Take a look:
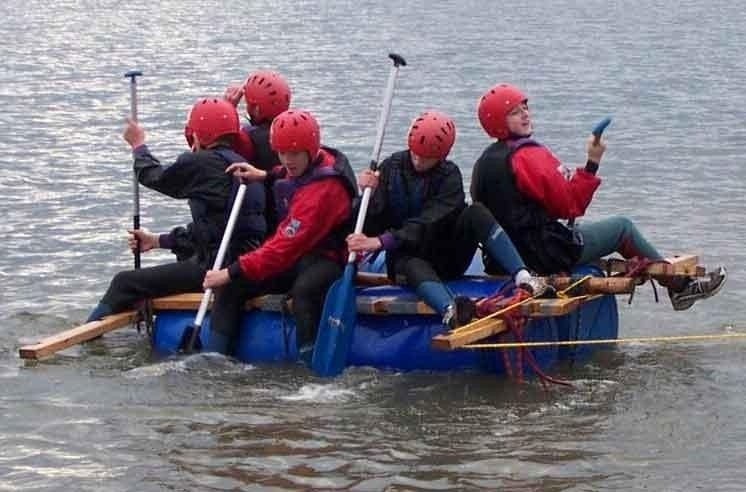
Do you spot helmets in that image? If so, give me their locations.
[477,84,528,140]
[408,111,456,162]
[269,110,320,163]
[185,98,239,147]
[245,73,290,122]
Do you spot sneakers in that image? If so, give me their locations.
[513,276,555,298]
[444,295,475,330]
[670,266,727,311]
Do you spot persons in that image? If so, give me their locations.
[85,94,267,323]
[224,70,291,235]
[202,109,360,344]
[345,108,558,332]
[469,84,728,311]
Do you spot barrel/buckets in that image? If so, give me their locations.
[150,254,619,376]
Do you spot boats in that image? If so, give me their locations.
[19,243,711,379]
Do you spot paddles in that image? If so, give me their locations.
[312,55,405,380]
[124,71,144,269]
[176,167,250,358]
